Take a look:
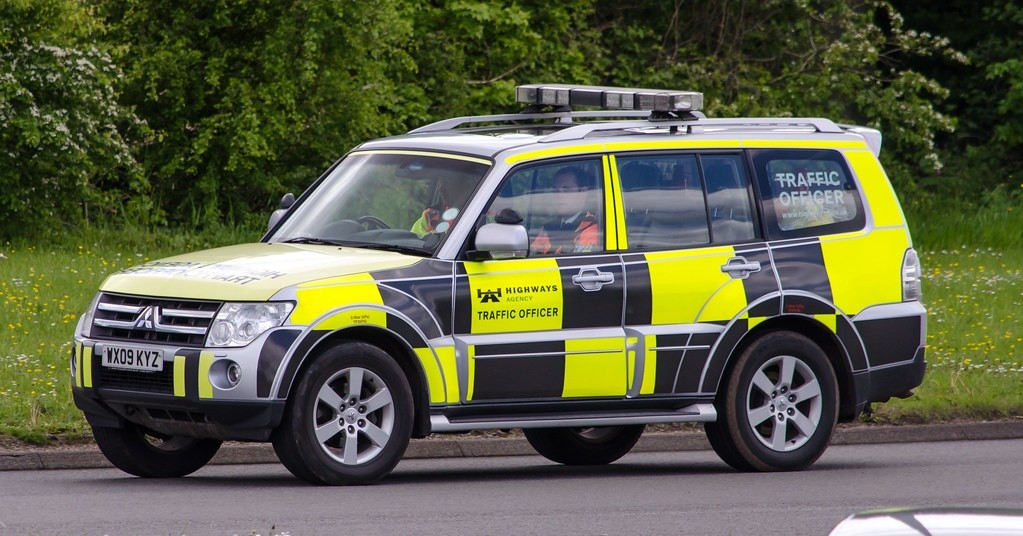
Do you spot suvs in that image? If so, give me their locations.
[71,83,926,489]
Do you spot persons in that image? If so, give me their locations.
[411,178,497,239]
[531,167,599,254]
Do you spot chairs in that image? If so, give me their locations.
[620,160,664,250]
[701,156,741,191]
